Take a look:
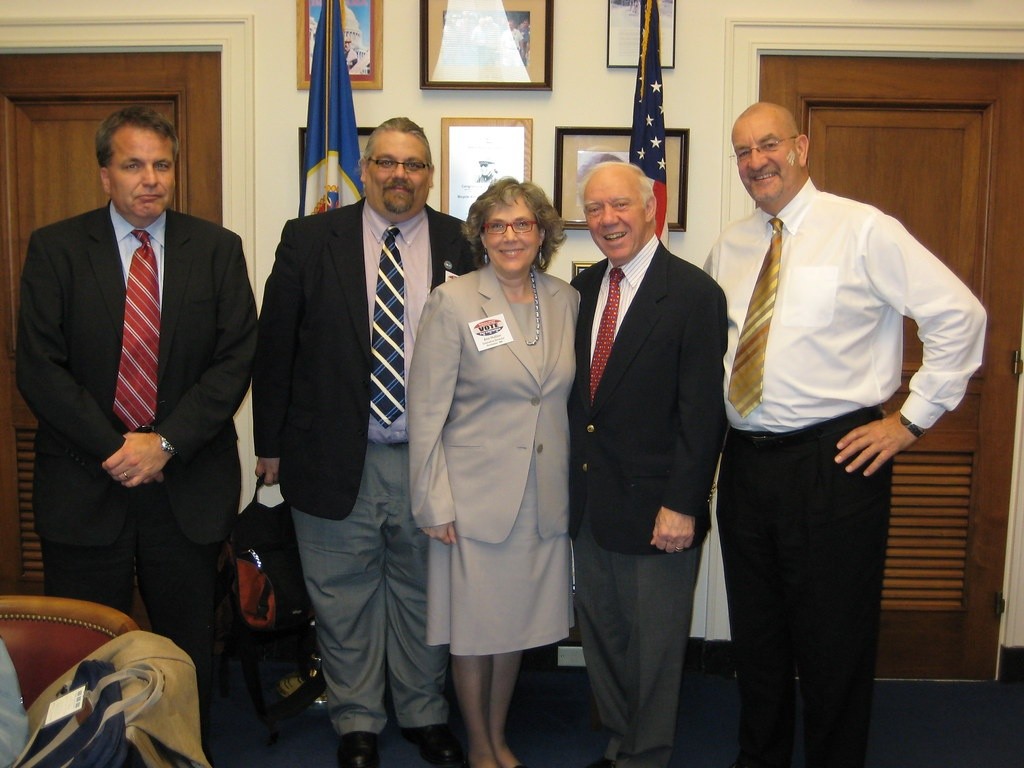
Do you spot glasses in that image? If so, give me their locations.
[736,134,799,160]
[367,155,430,176]
[482,219,537,235]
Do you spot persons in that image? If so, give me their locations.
[404,178,580,768]
[14,105,258,768]
[566,162,728,768]
[343,37,357,66]
[250,117,486,768]
[704,101,988,768]
[470,15,530,64]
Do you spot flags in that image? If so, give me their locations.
[629,0,668,252]
[298,0,362,218]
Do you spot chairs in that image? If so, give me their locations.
[0,594,212,768]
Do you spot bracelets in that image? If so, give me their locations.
[900,415,926,438]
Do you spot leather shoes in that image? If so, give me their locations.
[403,721,466,767]
[337,731,381,768]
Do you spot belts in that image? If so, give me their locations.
[732,405,885,447]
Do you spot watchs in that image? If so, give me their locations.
[160,436,174,453]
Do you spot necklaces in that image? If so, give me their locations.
[524,272,540,346]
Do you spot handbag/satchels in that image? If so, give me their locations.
[3,659,163,768]
[219,475,329,716]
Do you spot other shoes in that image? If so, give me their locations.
[586,756,617,768]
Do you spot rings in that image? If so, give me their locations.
[123,472,130,479]
[675,547,683,552]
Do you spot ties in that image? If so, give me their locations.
[365,227,406,428]
[114,229,160,435]
[727,216,783,419]
[589,267,624,406]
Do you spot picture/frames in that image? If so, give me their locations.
[606,0,676,69]
[296,0,384,91]
[298,127,378,218]
[419,0,554,91]
[440,117,533,224]
[572,261,597,279]
[554,125,690,233]
